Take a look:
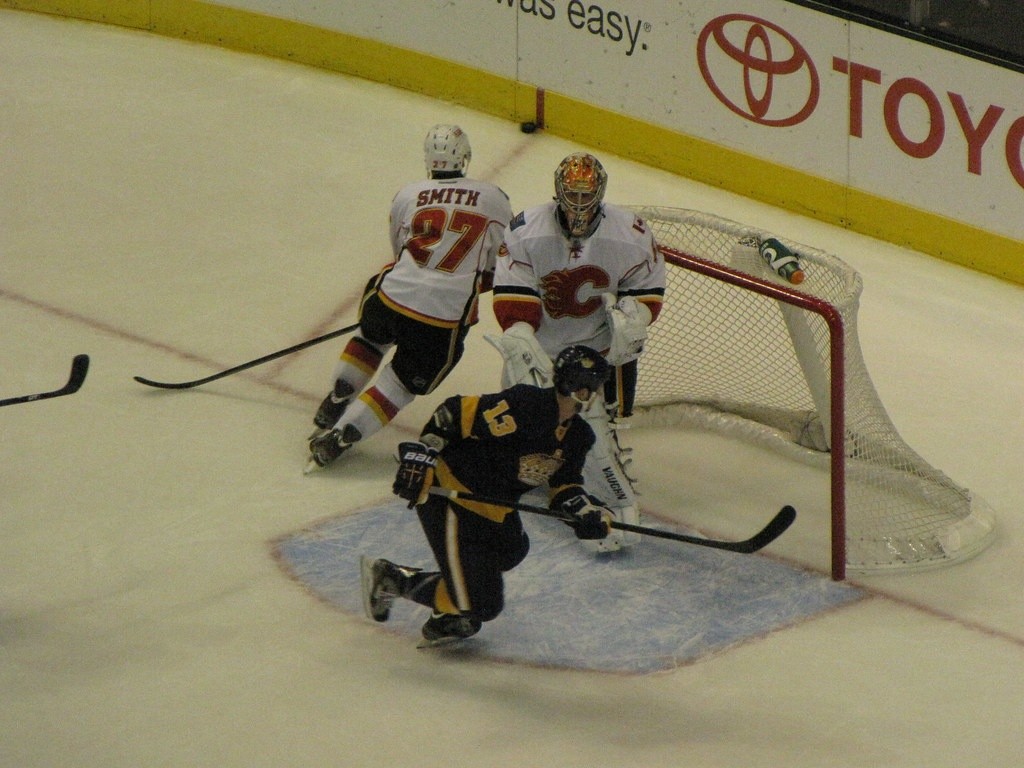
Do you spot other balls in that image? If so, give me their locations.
[520,121,537,133]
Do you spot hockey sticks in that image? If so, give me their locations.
[427,486,797,554]
[132,323,360,391]
[0,353,90,407]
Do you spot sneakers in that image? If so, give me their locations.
[304,424,362,473]
[415,610,482,649]
[308,379,355,440]
[359,554,423,626]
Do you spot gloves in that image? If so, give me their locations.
[393,443,440,511]
[550,486,611,540]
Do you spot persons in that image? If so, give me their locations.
[358,345,617,650]
[482,153,668,554]
[303,124,512,474]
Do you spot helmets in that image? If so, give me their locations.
[555,345,609,393]
[424,125,472,172]
[555,152,607,234]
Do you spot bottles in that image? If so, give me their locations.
[758,239,805,284]
